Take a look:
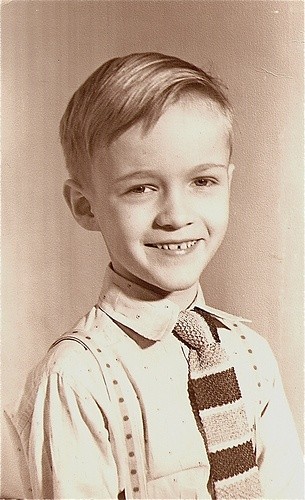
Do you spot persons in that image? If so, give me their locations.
[16,51,304,499]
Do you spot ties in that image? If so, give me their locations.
[174,310,263,499]
